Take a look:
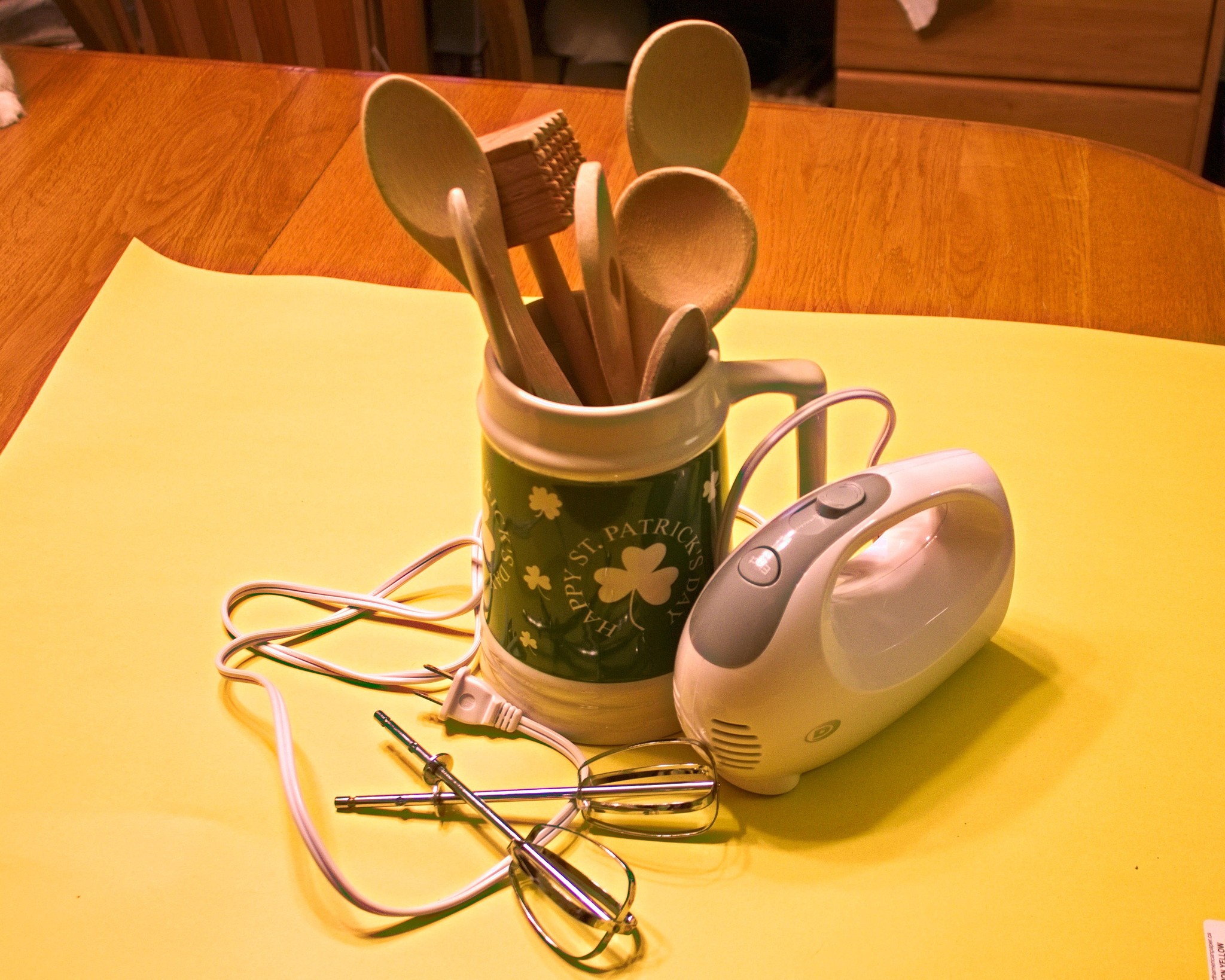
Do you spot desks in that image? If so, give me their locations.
[0,45,1225,980]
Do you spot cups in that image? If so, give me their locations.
[479,288,828,746]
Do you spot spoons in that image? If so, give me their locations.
[614,166,758,392]
[625,19,751,178]
[360,74,583,407]
